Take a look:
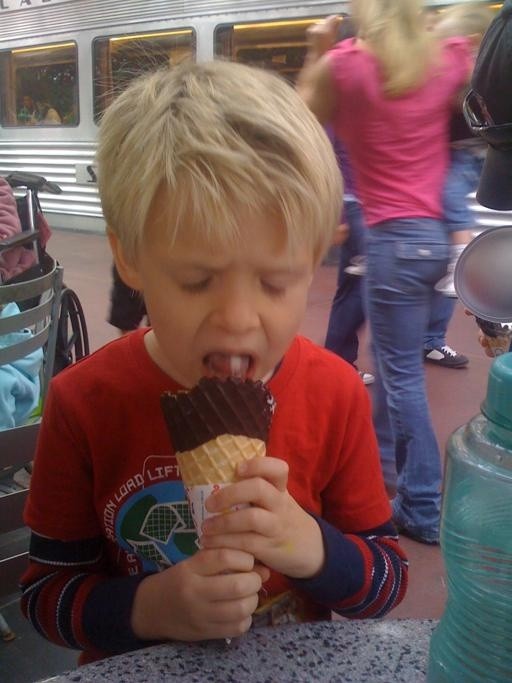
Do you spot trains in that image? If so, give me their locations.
[0,0,512,272]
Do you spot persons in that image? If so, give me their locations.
[15,93,42,126]
[321,13,375,384]
[294,0,452,552]
[25,93,62,126]
[103,264,150,336]
[424,137,490,367]
[18,52,413,666]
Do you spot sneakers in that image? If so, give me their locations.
[424,344,469,368]
[357,370,376,385]
[389,516,440,545]
[345,256,368,277]
[434,246,467,299]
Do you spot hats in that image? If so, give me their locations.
[461,0,511,212]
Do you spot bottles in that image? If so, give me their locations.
[418,346,512,682]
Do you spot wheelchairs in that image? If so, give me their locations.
[0,170,91,371]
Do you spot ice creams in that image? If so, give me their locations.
[473,314,512,358]
[159,374,277,646]
